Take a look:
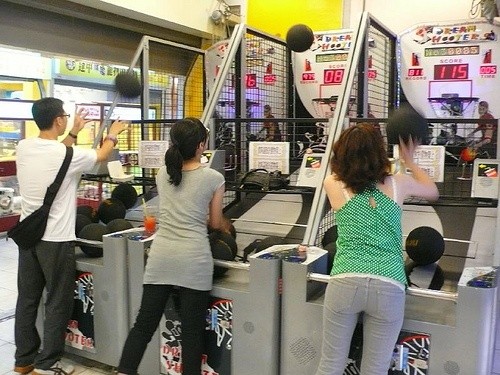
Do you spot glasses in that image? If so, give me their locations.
[58,114,70,118]
[206,128,211,134]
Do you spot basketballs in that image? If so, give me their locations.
[208,218,238,278]
[255,235,288,253]
[286,23,314,53]
[386,108,428,148]
[404,226,446,265]
[73,183,139,259]
[321,225,339,263]
[115,70,143,98]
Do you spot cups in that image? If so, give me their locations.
[145,216,156,232]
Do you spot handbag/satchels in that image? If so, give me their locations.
[234,167,291,192]
[6,205,50,250]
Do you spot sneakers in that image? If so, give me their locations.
[15,363,34,374]
[32,359,75,375]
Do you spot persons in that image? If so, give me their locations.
[316,122,440,375]
[257,105,281,141]
[14,98,129,375]
[116,117,230,375]
[467,101,497,157]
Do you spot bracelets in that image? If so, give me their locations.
[104,135,118,147]
[69,133,77,138]
